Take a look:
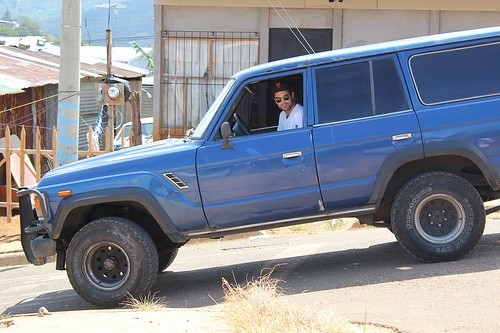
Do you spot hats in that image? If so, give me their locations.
[270,80,291,94]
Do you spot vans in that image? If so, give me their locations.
[113,117,154,152]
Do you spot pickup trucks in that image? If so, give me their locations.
[17,25,500,307]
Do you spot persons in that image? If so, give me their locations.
[272,80,305,132]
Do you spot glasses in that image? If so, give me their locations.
[272,93,291,102]
